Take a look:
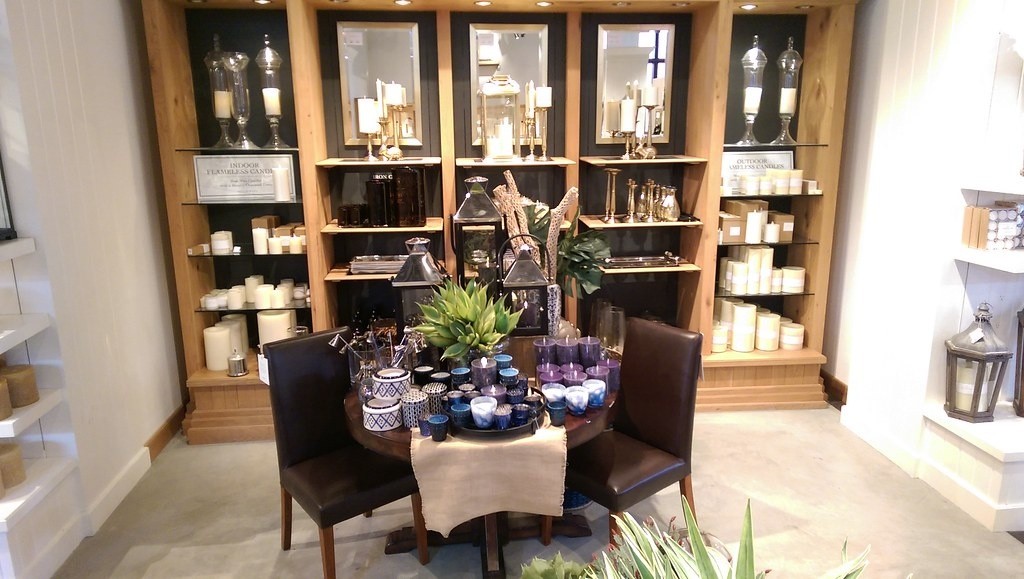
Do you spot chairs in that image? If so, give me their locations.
[262,325,430,579]
[539,315,704,552]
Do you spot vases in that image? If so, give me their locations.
[448,344,503,373]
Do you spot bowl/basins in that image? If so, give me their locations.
[360,397,403,432]
[371,368,412,400]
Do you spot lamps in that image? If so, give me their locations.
[711,297,805,353]
[521,33,524,37]
[513,33,519,40]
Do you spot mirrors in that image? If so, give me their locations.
[469,23,549,147]
[596,23,675,146]
[336,20,423,147]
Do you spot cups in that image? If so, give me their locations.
[548,401,567,427]
[442,396,448,411]
[583,379,606,408]
[470,396,512,430]
[358,379,374,403]
[499,368,520,390]
[542,383,566,404]
[418,414,432,436]
[431,372,451,391]
[451,404,470,427]
[565,386,589,416]
[428,415,449,442]
[524,395,544,419]
[494,355,513,370]
[415,366,434,388]
[514,404,530,426]
[447,390,481,411]
[451,368,475,394]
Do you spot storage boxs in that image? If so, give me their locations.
[969,207,989,249]
[802,179,818,192]
[962,206,975,247]
[978,206,1018,251]
[719,199,796,243]
[349,342,386,387]
[251,215,307,252]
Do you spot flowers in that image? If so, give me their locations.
[410,274,525,362]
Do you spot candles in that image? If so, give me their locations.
[764,220,780,243]
[213,88,233,118]
[779,86,797,114]
[605,98,620,131]
[252,227,269,255]
[986,204,1024,251]
[620,100,637,132]
[375,80,383,119]
[634,84,638,90]
[630,90,634,99]
[0,355,39,421]
[718,245,807,294]
[742,86,762,114]
[738,167,803,196]
[202,314,249,371]
[524,83,529,115]
[262,87,282,116]
[0,443,27,501]
[533,335,621,418]
[486,137,500,155]
[471,357,508,429]
[268,235,284,255]
[358,99,379,134]
[634,90,641,106]
[382,83,388,118]
[271,163,291,202]
[256,310,298,354]
[745,208,762,243]
[210,230,234,254]
[535,83,553,108]
[402,87,407,107]
[653,77,665,108]
[626,84,630,97]
[641,86,659,105]
[386,84,403,107]
[199,275,311,308]
[289,233,302,254]
[495,124,512,154]
[528,80,535,115]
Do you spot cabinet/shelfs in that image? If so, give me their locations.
[139,0,859,449]
[920,31,1024,467]
[0,239,78,532]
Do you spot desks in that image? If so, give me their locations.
[342,336,621,579]
